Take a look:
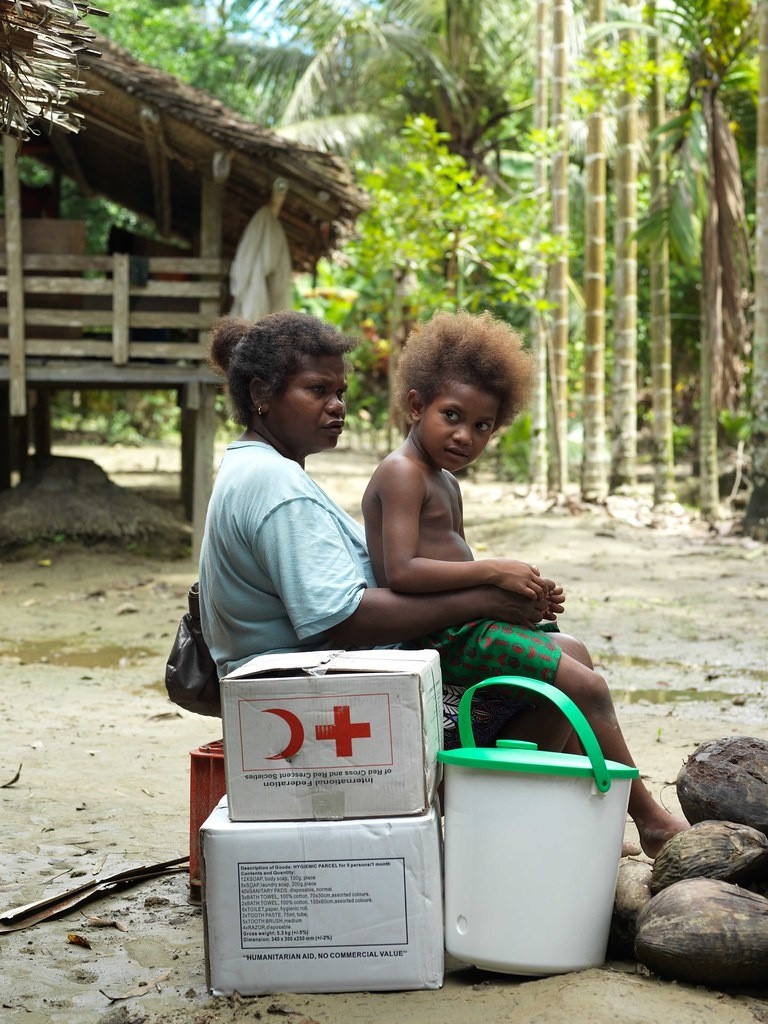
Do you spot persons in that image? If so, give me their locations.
[198,314,641,858]
[361,309,691,858]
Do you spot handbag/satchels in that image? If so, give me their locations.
[165,582,222,718]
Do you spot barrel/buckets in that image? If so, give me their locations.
[436,673,637,976]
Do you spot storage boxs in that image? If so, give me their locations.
[192,648,447,998]
